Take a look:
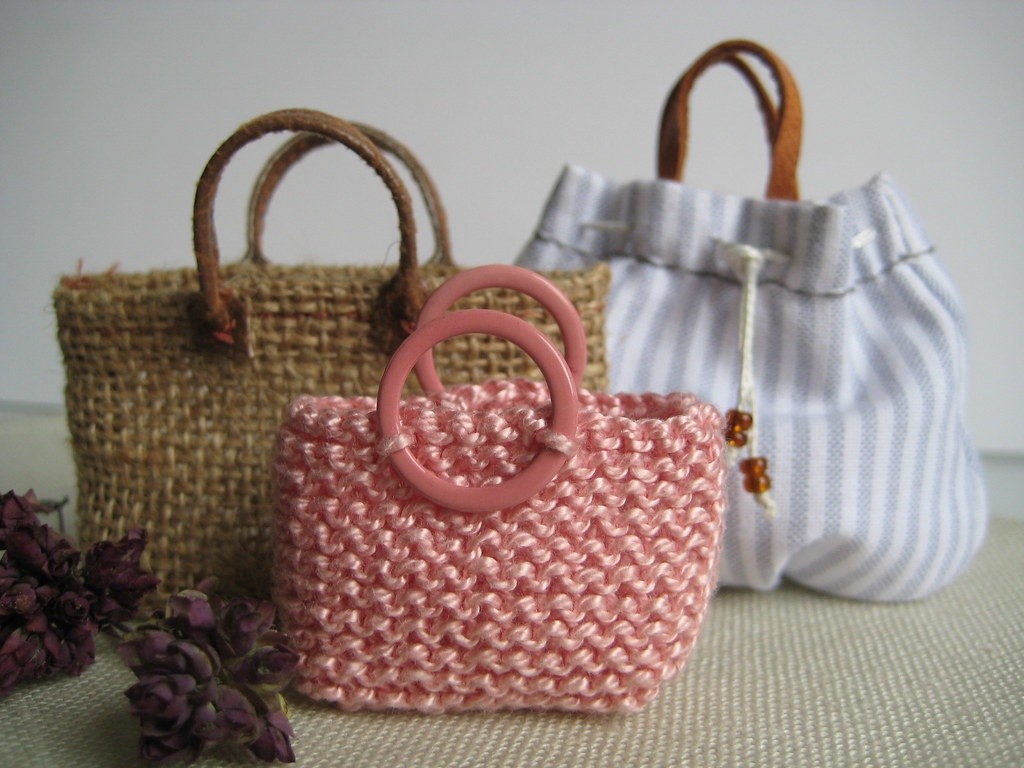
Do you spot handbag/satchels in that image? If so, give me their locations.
[512,39,988,604]
[52,108,612,600]
[270,265,727,715]
